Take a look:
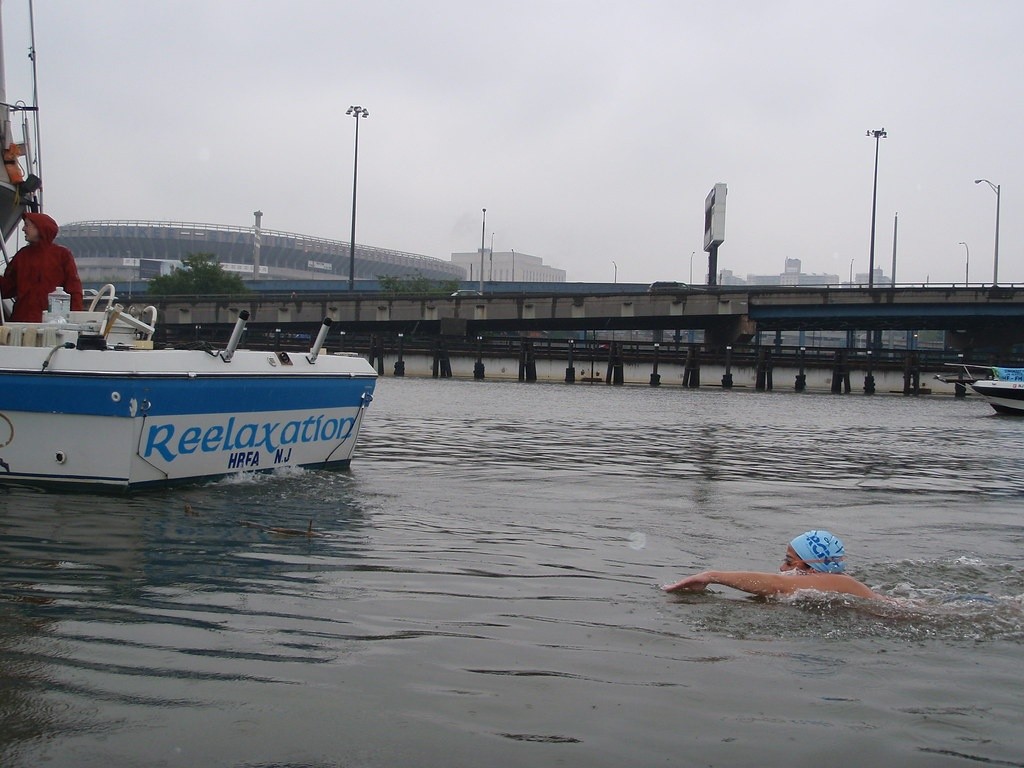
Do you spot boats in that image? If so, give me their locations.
[944,363,1024,416]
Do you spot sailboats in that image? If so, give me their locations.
[0,1,379,496]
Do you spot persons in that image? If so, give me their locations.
[0,213,84,323]
[665,530,875,597]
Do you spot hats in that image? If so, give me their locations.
[791,530,846,574]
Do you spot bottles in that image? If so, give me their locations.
[48,286,71,315]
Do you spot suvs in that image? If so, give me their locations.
[647,280,700,297]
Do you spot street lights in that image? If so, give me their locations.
[865,127,886,286]
[850,258,854,289]
[345,106,370,288]
[612,260,617,283]
[512,248,514,281]
[479,208,486,292]
[891,212,898,290]
[690,252,696,285]
[975,180,1001,286]
[959,242,969,287]
[491,233,494,279]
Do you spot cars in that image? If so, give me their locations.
[451,290,484,297]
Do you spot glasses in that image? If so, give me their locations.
[785,555,794,567]
